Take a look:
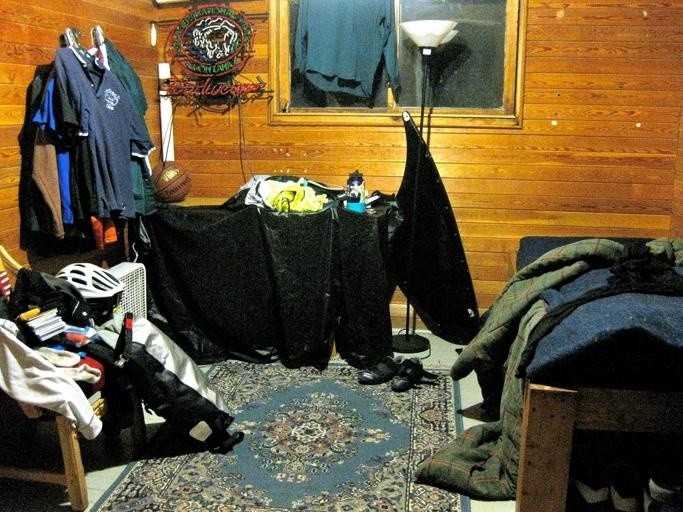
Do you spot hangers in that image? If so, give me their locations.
[33,24,136,58]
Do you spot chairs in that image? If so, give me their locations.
[0,246,151,511]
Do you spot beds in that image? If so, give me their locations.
[503,225,683,512]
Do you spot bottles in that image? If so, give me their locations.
[346,170,365,215]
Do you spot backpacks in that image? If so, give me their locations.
[112,312,244,454]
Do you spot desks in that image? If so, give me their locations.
[157,185,409,366]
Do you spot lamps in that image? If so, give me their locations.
[392,17,460,353]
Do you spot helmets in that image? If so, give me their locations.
[53,261,124,326]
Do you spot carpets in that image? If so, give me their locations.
[92,362,472,512]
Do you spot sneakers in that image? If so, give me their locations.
[391,357,423,391]
[360,356,396,385]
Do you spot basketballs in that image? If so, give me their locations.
[154,162,188,201]
